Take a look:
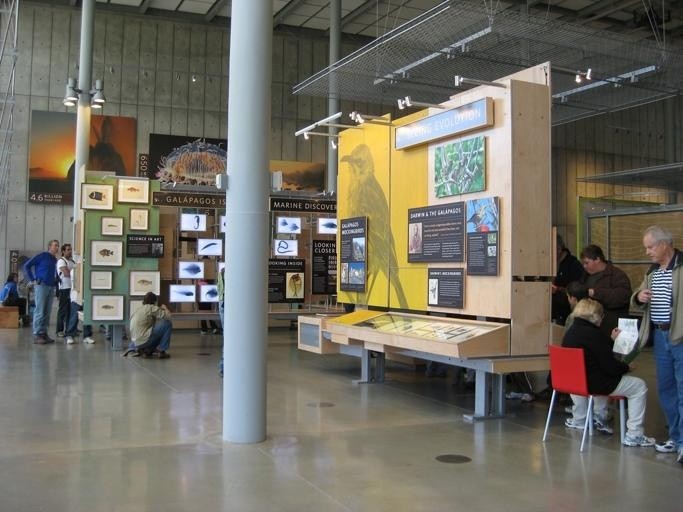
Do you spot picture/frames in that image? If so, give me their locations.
[78,174,162,323]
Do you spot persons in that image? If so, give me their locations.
[504,228,634,419]
[128,292,171,358]
[1,239,92,343]
[215,267,225,376]
[561,296,656,448]
[626,225,683,465]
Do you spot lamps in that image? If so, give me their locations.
[61,74,107,111]
[304,75,507,151]
[529,0,666,106]
[572,64,638,88]
[373,0,499,86]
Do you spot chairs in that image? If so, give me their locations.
[536,343,630,455]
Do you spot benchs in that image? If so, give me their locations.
[0,301,19,329]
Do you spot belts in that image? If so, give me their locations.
[654,323,672,331]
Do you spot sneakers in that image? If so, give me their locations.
[592,414,615,434]
[158,351,169,360]
[654,440,676,452]
[34,332,96,344]
[624,433,656,447]
[565,416,585,430]
